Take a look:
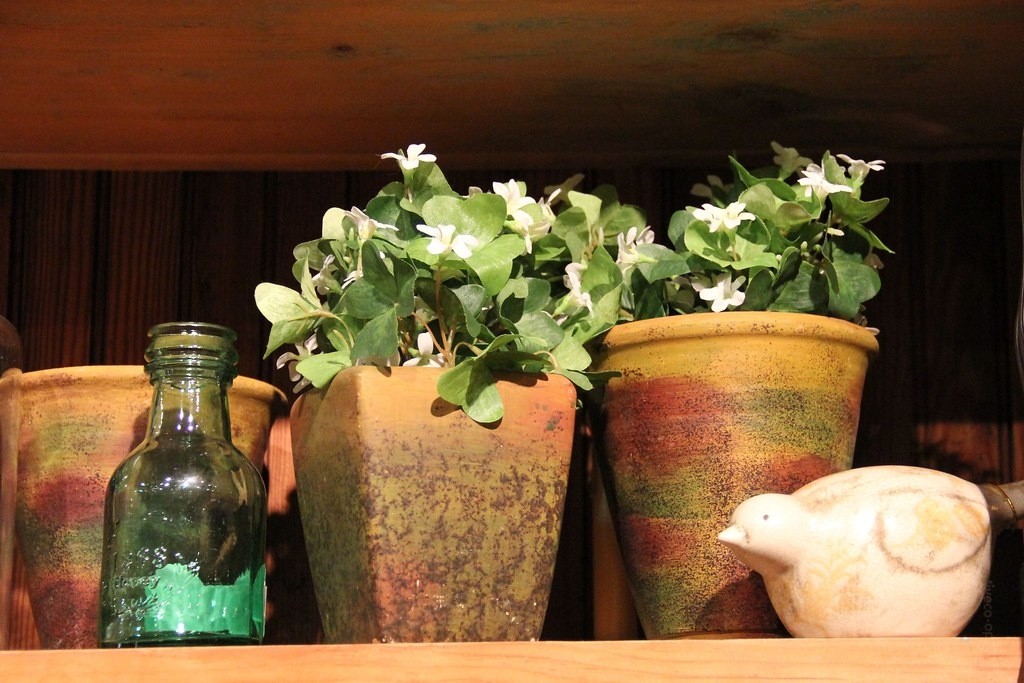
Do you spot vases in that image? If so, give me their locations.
[18,363,287,648]
[585,312,879,639]
[289,364,577,644]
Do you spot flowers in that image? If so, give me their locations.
[254,142,898,424]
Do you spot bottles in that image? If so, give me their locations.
[98,322,269,647]
